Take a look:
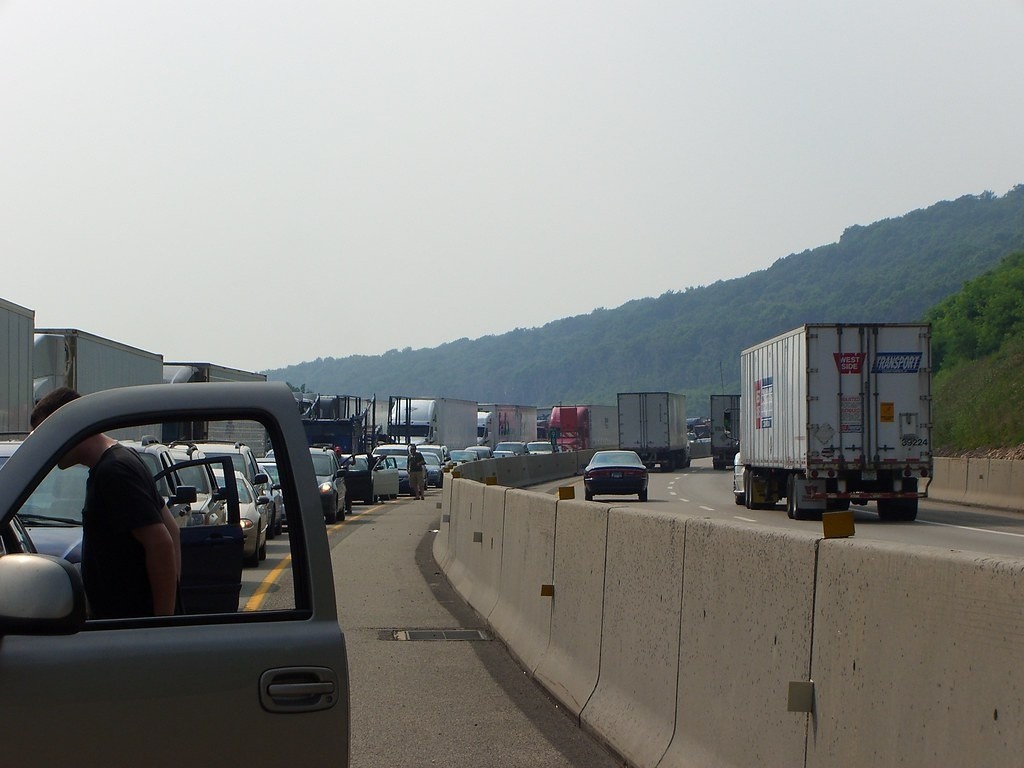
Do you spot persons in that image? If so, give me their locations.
[406,444,426,500]
[334,446,356,515]
[367,452,387,504]
[28,385,180,620]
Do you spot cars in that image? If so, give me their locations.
[580,451,652,504]
[492,450,516,458]
[464,446,491,460]
[0,380,478,768]
[685,416,712,443]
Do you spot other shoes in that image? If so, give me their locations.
[414,497,420,500]
[345,510,352,514]
[421,497,424,500]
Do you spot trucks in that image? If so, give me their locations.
[32,326,166,446]
[387,396,478,450]
[709,394,743,470]
[473,403,538,450]
[616,391,692,474]
[162,361,269,457]
[0,298,37,441]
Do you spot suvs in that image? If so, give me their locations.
[494,441,528,455]
[528,440,556,455]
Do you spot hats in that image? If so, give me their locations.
[334,446,341,451]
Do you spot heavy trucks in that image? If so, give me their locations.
[723,321,940,522]
[547,403,620,453]
